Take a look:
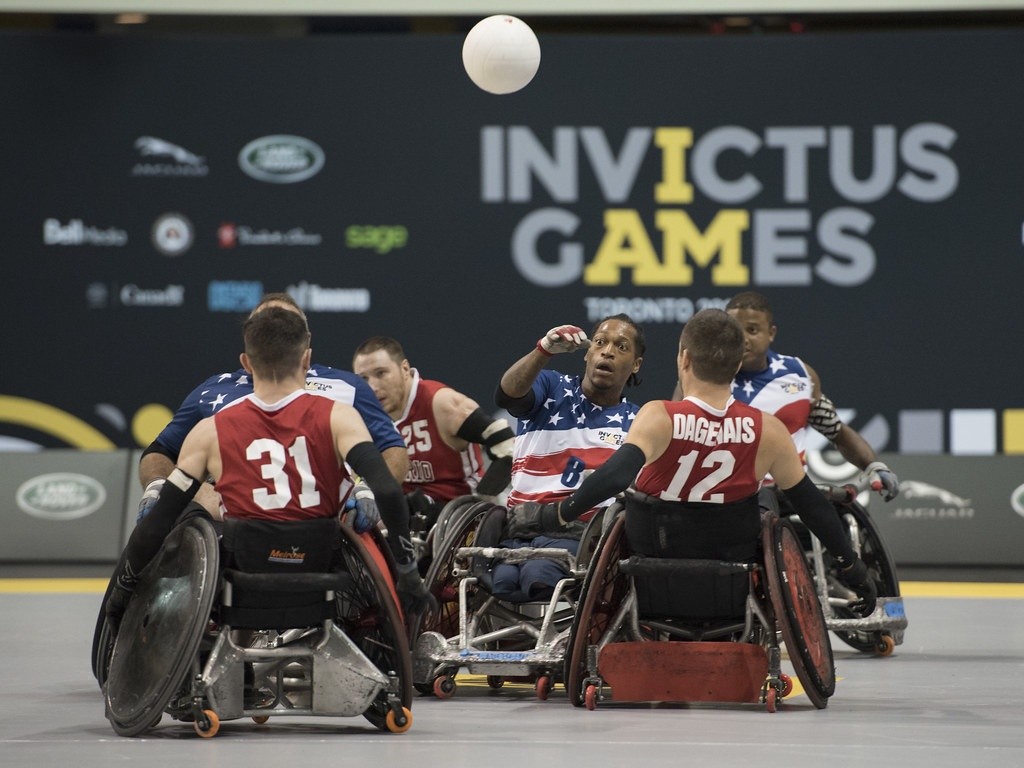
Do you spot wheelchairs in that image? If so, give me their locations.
[92,484,907,740]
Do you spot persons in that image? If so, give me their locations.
[134,286,913,708]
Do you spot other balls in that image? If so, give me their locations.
[462,14,542,96]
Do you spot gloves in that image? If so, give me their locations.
[865,462,899,502]
[105,559,138,642]
[537,325,592,357]
[504,502,574,538]
[841,558,877,618]
[137,478,166,524]
[395,557,439,616]
[345,479,382,534]
